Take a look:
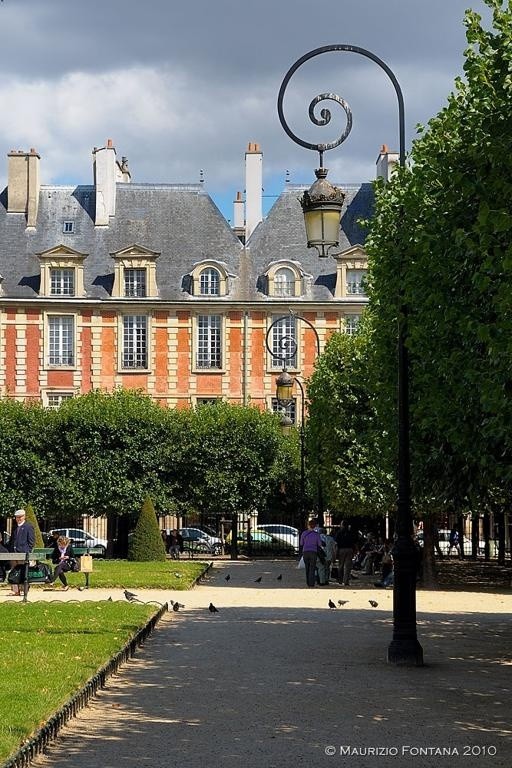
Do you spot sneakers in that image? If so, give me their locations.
[307,581,350,590]
[7,591,32,598]
[46,578,70,591]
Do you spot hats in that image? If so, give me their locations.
[14,508,26,516]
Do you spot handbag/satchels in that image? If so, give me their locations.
[80,550,94,572]
[66,557,80,571]
[295,555,305,570]
[8,563,52,584]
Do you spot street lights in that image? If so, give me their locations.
[268,39,433,667]
[259,309,331,527]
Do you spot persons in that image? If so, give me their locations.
[45,534,78,591]
[447,523,461,556]
[6,509,34,596]
[160,528,184,560]
[293,516,397,589]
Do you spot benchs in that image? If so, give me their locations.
[178,537,201,559]
[19,545,102,592]
[0,550,50,603]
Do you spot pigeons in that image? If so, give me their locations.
[277,574,283,579]
[208,602,220,613]
[368,600,378,608]
[338,600,350,608]
[107,596,113,602]
[171,599,185,608]
[173,602,180,613]
[328,599,337,610]
[125,589,138,598]
[224,574,230,581]
[124,591,138,602]
[254,576,262,584]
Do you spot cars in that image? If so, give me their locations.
[50,524,107,556]
[162,521,299,555]
[413,527,500,556]
